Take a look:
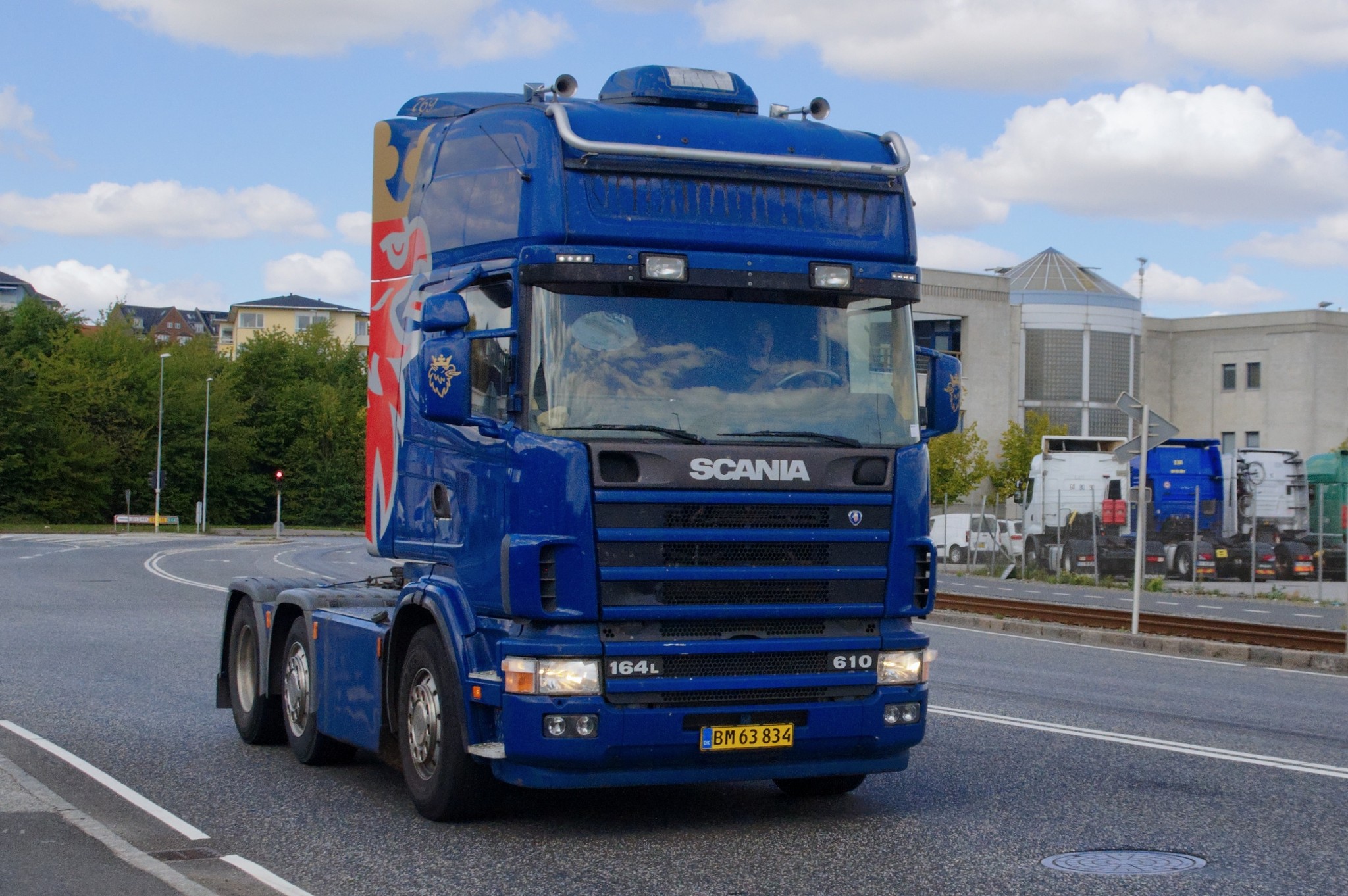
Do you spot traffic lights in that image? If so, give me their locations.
[276,471,284,491]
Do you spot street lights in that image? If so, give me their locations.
[154,352,173,533]
[202,377,213,533]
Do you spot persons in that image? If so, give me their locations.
[713,307,849,392]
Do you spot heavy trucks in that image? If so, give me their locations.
[1129,438,1348,582]
[210,63,962,825]
[1012,435,1171,586]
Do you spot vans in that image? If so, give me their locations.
[927,513,1003,565]
[995,518,1024,563]
